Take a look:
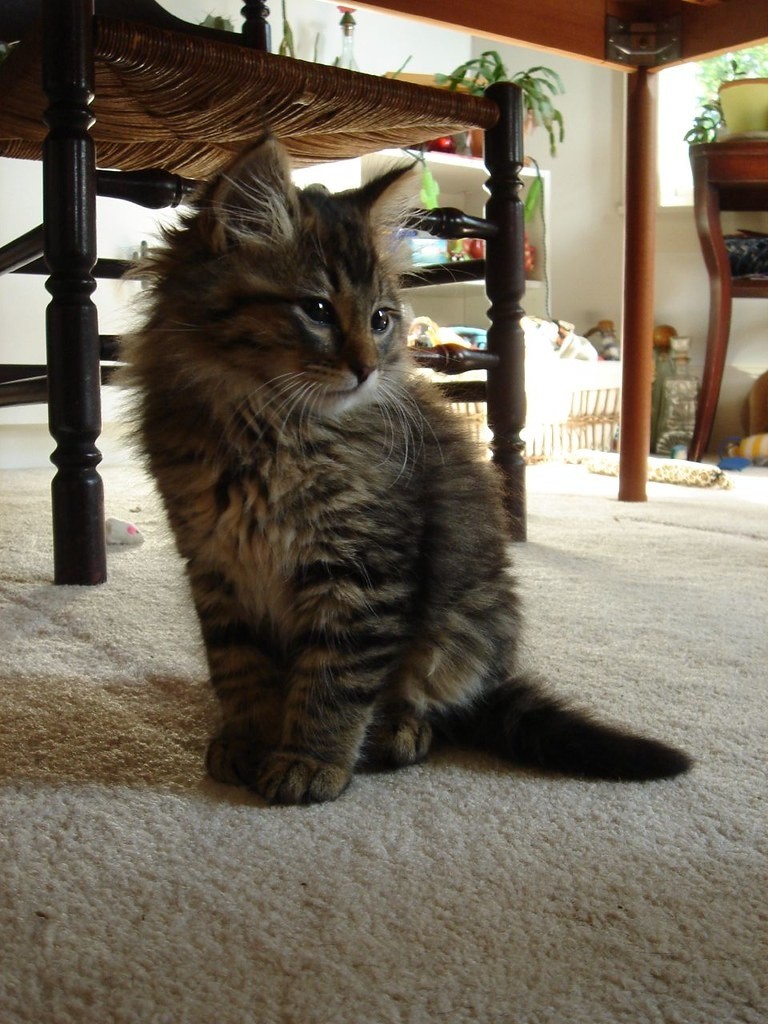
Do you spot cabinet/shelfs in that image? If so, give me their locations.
[360,147,553,334]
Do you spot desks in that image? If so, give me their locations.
[684,141,768,461]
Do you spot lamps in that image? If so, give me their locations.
[338,9,356,37]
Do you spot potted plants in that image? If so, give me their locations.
[685,60,768,143]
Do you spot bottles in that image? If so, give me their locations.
[654,337,701,454]
[727,433,768,460]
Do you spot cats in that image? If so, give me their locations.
[115,121,699,807]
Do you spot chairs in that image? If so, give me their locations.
[0,0,525,589]
